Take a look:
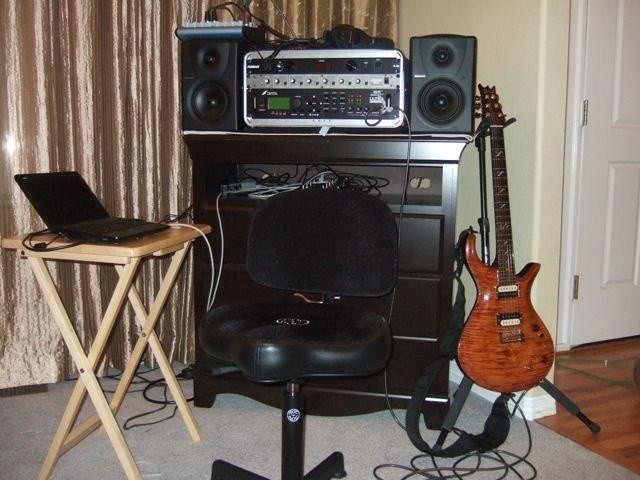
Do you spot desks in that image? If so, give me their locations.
[1,220,214,479]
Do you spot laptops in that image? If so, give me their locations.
[12,170,170,243]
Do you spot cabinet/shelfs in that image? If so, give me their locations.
[180,129,472,432]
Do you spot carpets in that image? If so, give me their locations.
[0,361,637,480]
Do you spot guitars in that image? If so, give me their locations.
[456,84,555,393]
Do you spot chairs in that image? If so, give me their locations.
[196,185,397,480]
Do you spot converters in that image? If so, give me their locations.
[219,181,258,194]
[180,364,193,382]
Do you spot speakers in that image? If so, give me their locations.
[181,40,246,131]
[408,33,478,133]
